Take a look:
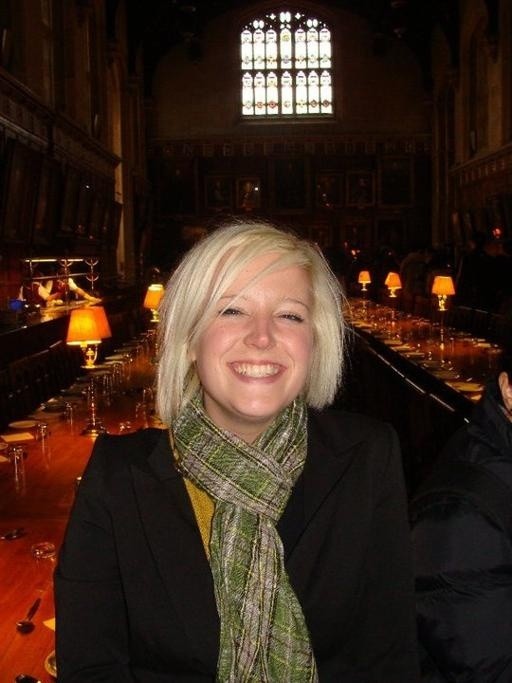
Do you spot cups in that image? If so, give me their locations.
[350,298,499,381]
[9,325,159,578]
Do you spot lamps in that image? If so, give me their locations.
[384,270,403,315]
[141,282,166,336]
[65,305,115,440]
[357,270,373,300]
[431,275,456,335]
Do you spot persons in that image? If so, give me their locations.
[408,344,512,683]
[18,257,124,306]
[323,245,511,346]
[51,214,412,683]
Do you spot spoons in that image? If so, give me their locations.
[0,527,24,541]
[16,599,43,632]
[15,674,41,683]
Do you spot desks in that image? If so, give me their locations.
[341,298,505,421]
[0,325,173,681]
